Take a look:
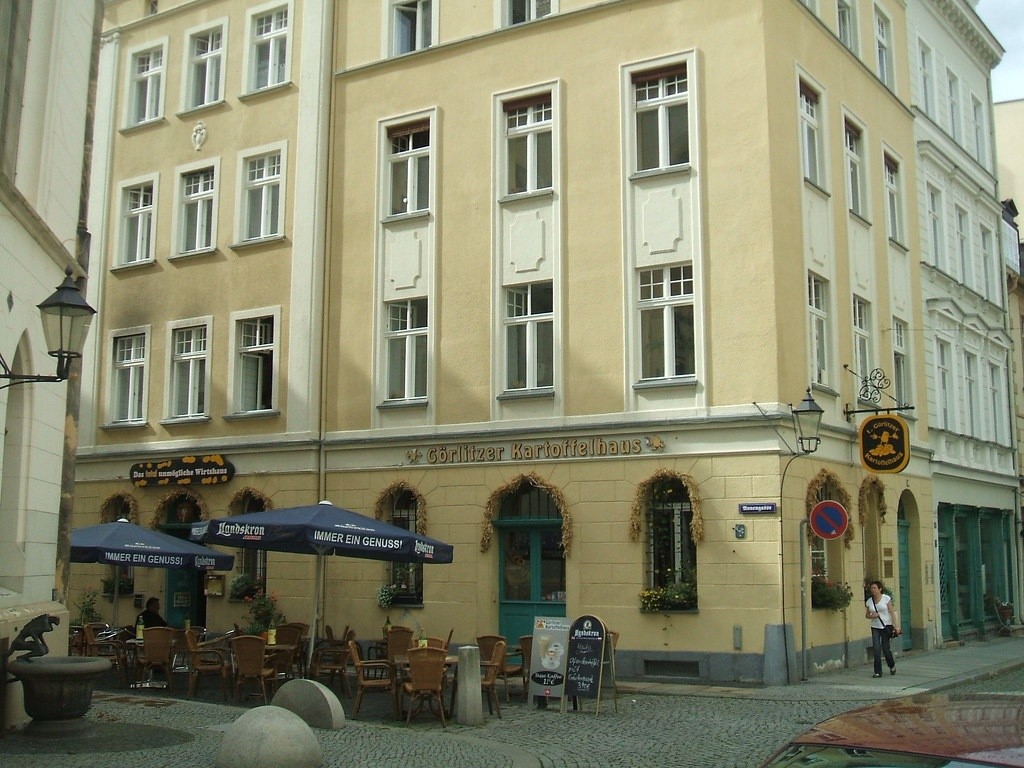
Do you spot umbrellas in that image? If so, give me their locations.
[189,500,454,679]
[69,518,235,640]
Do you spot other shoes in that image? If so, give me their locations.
[873,673,882,678]
[890,666,896,675]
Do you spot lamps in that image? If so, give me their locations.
[791,385,823,455]
[39,260,104,381]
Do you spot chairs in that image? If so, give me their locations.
[68,614,539,728]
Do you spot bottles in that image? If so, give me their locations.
[267,619,276,645]
[135,616,145,640]
[385,615,392,639]
[417,628,427,647]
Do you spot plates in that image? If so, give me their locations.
[548,647,564,657]
[541,659,560,669]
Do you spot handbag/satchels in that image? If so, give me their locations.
[885,625,899,638]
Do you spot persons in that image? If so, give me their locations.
[134,596,175,638]
[867,581,901,678]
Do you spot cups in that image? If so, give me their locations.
[537,634,551,657]
[552,642,562,652]
[545,650,557,665]
